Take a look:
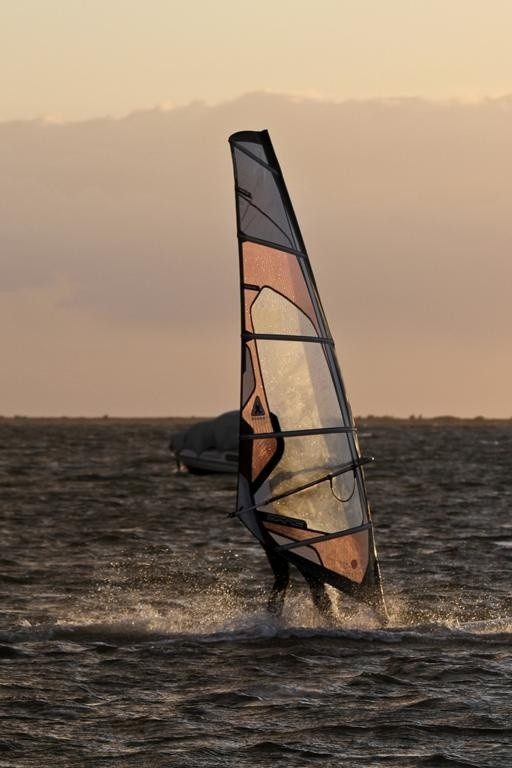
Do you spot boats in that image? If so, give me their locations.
[168,410,241,474]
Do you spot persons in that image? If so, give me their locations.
[265,551,336,625]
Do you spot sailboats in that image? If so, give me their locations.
[217,123,512,634]
